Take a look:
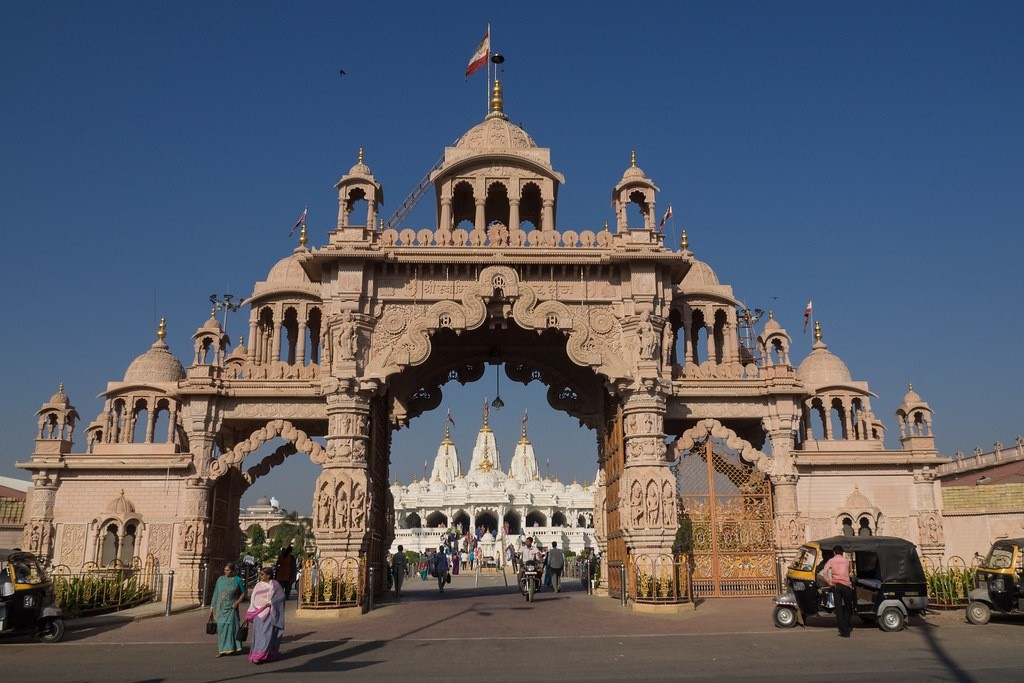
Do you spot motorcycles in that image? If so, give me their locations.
[0,547,65,644]
[511,554,545,603]
[965,538,1024,625]
[770,535,929,633]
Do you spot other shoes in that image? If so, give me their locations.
[440,588,443,592]
[838,633,850,638]
[253,660,258,664]
[215,653,225,658]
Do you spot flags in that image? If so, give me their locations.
[465,34,490,80]
[804,302,811,335]
[522,410,528,423]
[448,413,456,425]
[289,210,307,232]
[659,204,673,234]
[485,402,491,415]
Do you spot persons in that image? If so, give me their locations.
[243,551,253,564]
[211,562,245,658]
[823,546,855,638]
[452,553,460,576]
[392,545,409,598]
[547,541,564,593]
[434,545,450,592]
[244,567,286,666]
[517,537,544,591]
[417,550,438,580]
[542,551,552,585]
[437,520,510,565]
[534,521,539,527]
[275,545,296,599]
[460,549,474,571]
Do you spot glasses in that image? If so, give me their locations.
[259,573,266,576]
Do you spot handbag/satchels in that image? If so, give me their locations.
[235,619,249,642]
[206,612,217,635]
[447,570,451,583]
